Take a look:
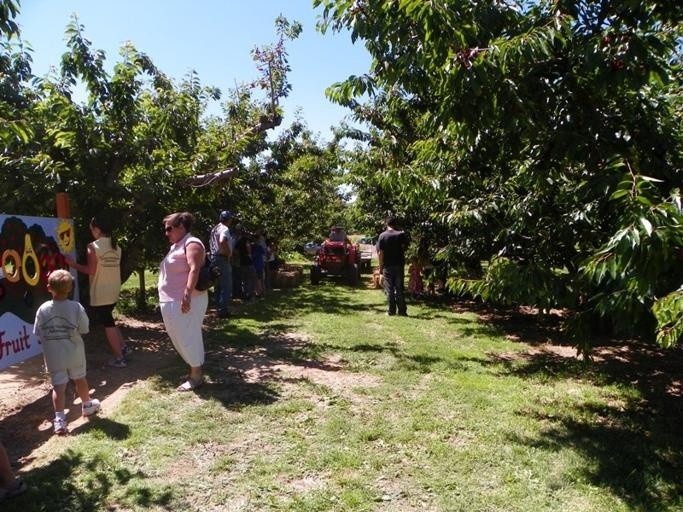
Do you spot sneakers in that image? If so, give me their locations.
[0,479,28,501]
[107,344,133,367]
[54,418,67,434]
[82,399,99,416]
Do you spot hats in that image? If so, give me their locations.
[220,211,232,219]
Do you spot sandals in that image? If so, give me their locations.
[177,376,202,391]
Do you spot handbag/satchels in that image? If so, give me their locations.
[196,262,224,291]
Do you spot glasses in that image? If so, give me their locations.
[164,226,172,231]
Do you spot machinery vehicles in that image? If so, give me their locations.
[310,239,373,286]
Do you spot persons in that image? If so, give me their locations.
[157,211,211,392]
[0,441,28,500]
[33,268,101,433]
[375,215,411,316]
[407,256,424,295]
[329,222,352,247]
[65,210,134,368]
[231,223,283,299]
[209,209,233,317]
